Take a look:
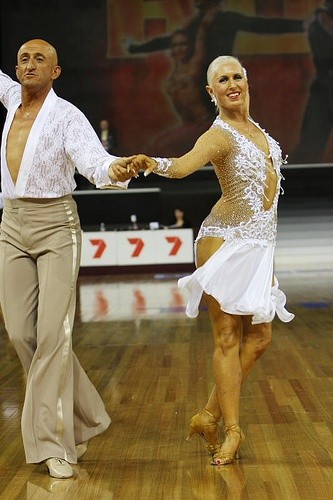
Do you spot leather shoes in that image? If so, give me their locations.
[46,457,73,479]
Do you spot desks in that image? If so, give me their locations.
[76,274,198,324]
[78,224,196,276]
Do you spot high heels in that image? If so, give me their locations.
[211,422,245,464]
[186,408,222,456]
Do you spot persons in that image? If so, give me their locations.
[0,39,139,478]
[127,56,294,465]
[94,119,117,157]
[169,207,192,228]
[118,0,333,167]
